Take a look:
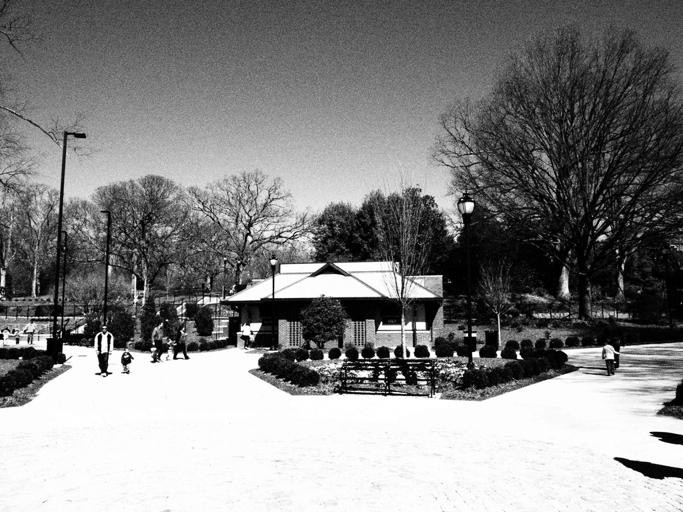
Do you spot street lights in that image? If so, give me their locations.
[456,191,476,336]
[220,256,228,279]
[267,250,279,339]
[100,210,111,325]
[52,130,85,338]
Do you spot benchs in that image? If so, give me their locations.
[337,359,436,398]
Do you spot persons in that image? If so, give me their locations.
[165,338,177,360]
[14,329,20,344]
[240,321,252,350]
[22,320,38,345]
[121,347,134,374]
[2,327,12,346]
[152,321,164,360]
[93,325,115,377]
[602,338,620,376]
[172,323,190,360]
